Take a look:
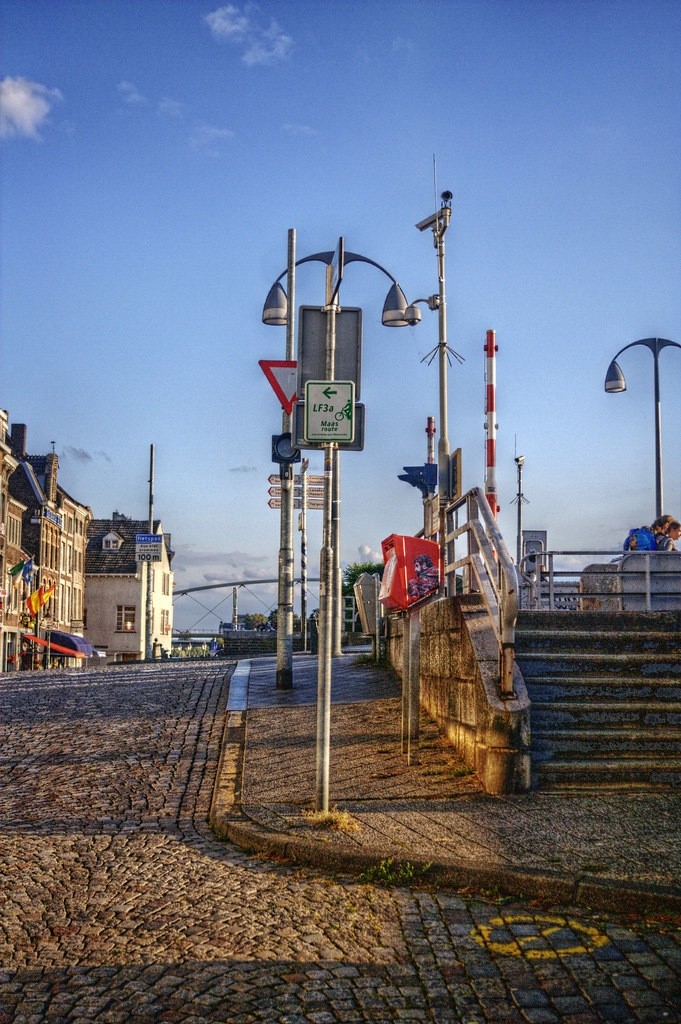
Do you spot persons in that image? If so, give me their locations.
[650,514,681,551]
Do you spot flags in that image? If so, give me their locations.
[10,559,25,589]
[22,558,34,586]
[26,585,54,617]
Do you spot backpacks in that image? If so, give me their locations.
[623,526,665,551]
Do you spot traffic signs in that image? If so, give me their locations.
[268,496,324,509]
[268,474,325,485]
[267,485,324,498]
[304,380,356,443]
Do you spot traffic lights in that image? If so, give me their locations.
[272,431,302,464]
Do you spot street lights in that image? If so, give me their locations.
[261,234,411,812]
[604,337,681,520]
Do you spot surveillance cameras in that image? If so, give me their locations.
[403,303,422,326]
[515,456,525,463]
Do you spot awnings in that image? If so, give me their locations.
[24,635,85,659]
[43,630,93,658]
